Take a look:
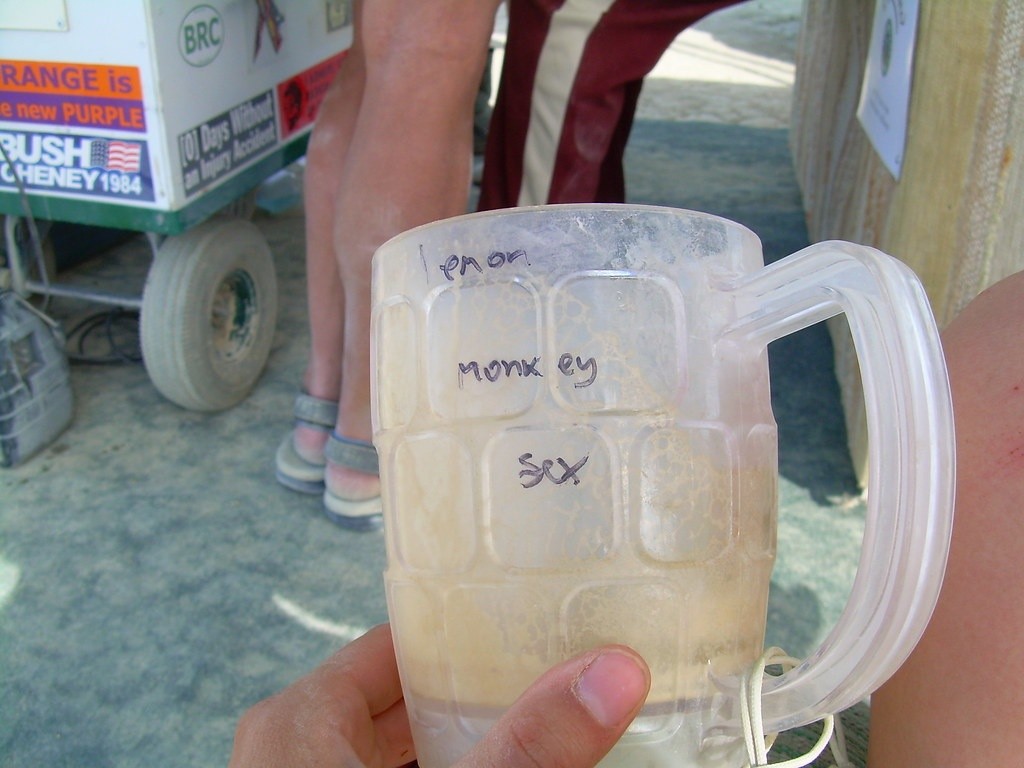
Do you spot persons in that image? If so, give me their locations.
[470,1,764,280]
[218,271,1024,767]
[273,0,505,535]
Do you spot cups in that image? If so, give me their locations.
[366,204,955,768]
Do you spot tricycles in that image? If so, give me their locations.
[0,0,500,414]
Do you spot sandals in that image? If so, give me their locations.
[323,432,382,533]
[273,390,338,496]
